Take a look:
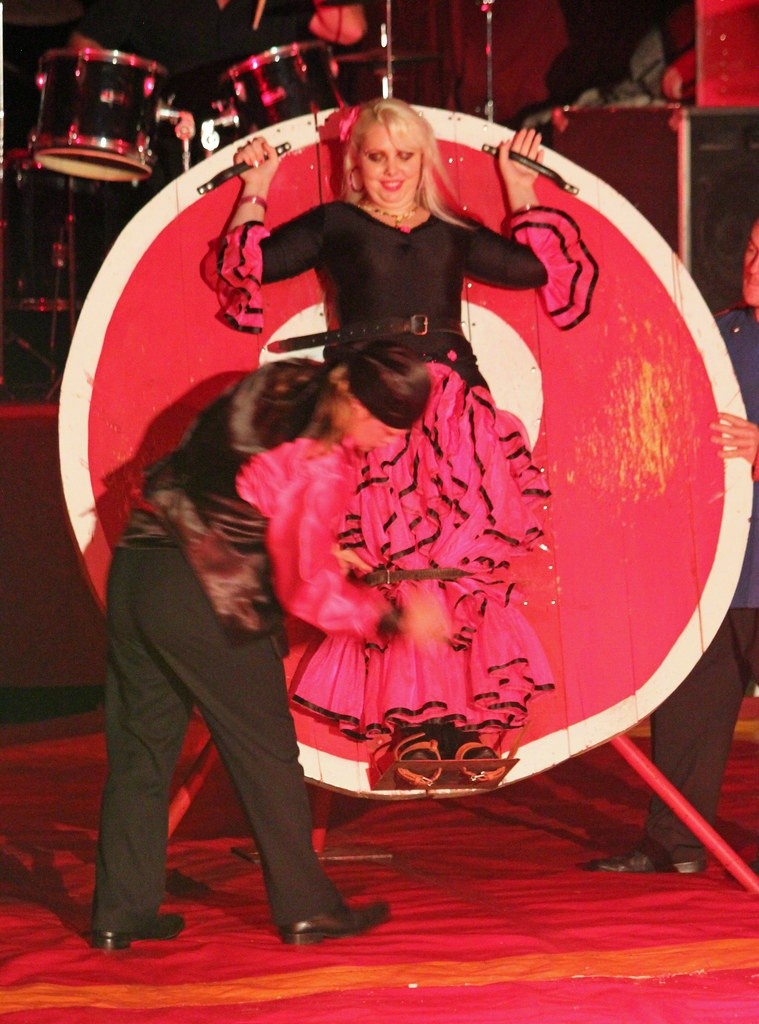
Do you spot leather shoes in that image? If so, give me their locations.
[589,844,705,873]
[92,915,183,950]
[281,903,390,945]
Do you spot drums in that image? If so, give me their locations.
[212,38,350,144]
[1,146,130,315]
[28,44,169,183]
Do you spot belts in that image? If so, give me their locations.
[267,314,465,354]
[118,526,263,554]
[361,566,471,584]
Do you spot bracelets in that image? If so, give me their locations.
[513,205,539,210]
[236,195,267,210]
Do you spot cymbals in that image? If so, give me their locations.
[3,0,84,28]
[334,44,441,66]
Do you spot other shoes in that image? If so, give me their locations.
[443,722,499,771]
[399,724,440,760]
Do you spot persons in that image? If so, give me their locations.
[590,220,759,874]
[92,343,431,949]
[218,98,600,786]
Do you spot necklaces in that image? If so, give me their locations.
[362,200,422,233]
